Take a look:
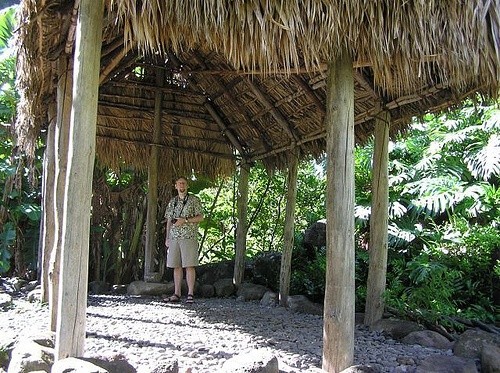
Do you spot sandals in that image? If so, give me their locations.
[163,294,181,302]
[187,294,193,303]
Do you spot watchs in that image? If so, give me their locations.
[185,217,189,222]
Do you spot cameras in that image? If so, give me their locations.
[172,219,178,224]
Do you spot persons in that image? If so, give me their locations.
[163,176,204,303]
